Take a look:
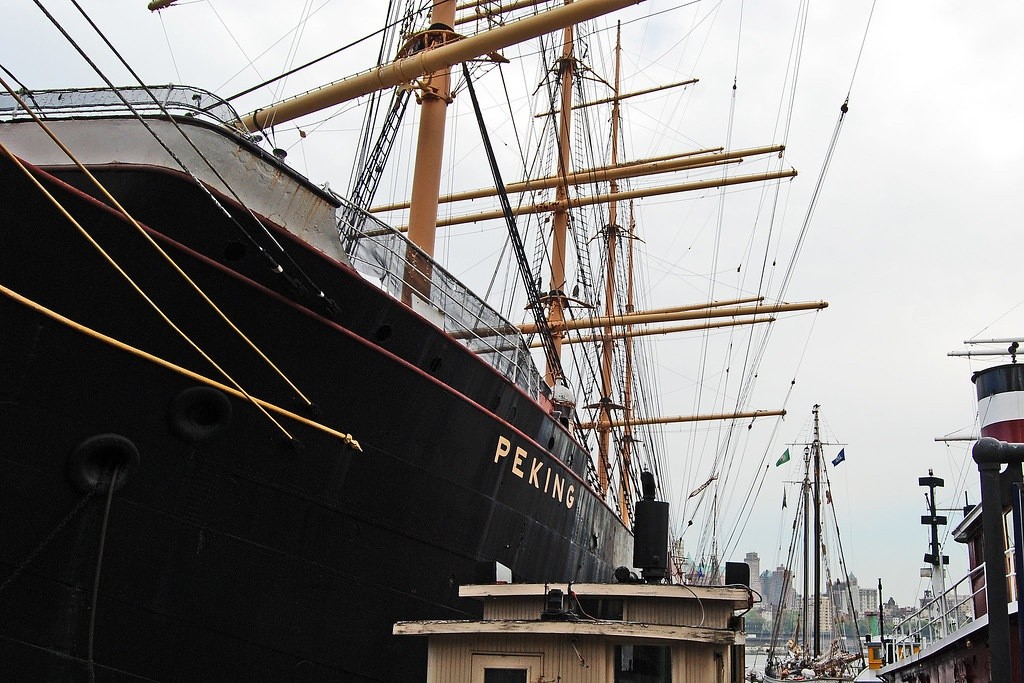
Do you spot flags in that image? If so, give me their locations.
[776,448,790,467]
[832,447,845,467]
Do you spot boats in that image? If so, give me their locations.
[878,334,1023,683]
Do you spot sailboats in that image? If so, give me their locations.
[0,0,831,683]
[748,402,869,683]
